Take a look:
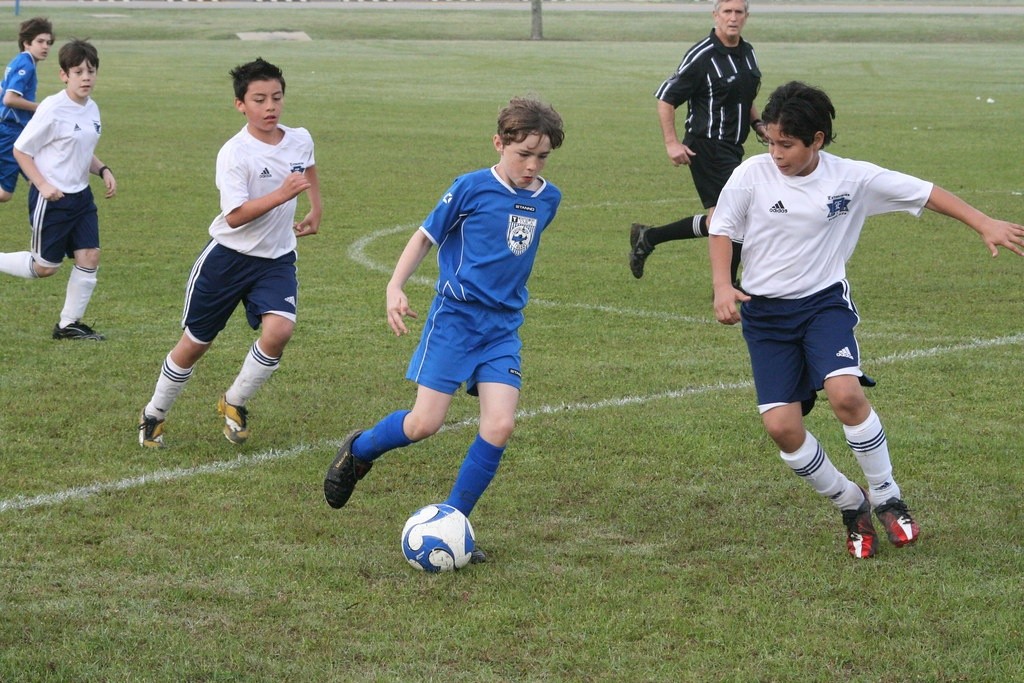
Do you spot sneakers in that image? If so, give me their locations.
[324,430,373,509]
[874,497,920,548]
[217,392,248,445]
[629,223,655,279]
[713,279,746,302]
[53,321,105,341]
[840,483,880,559]
[470,547,486,564]
[138,406,167,450]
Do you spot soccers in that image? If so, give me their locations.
[400,502,477,575]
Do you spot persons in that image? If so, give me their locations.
[708,81,1024,560]
[324,95,565,565]
[138,57,322,450]
[0,18,54,202]
[0,37,117,341]
[629,0,768,303]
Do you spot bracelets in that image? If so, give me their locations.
[99,166,112,179]
[751,119,764,131]
